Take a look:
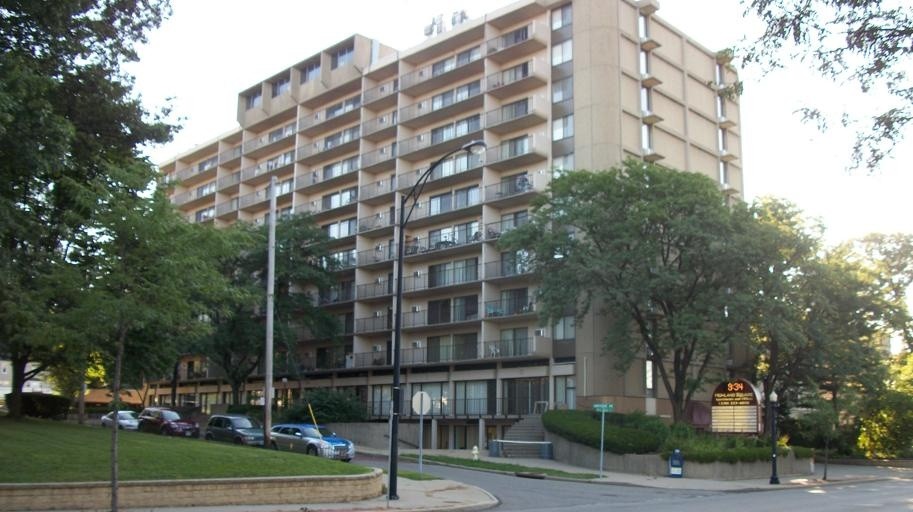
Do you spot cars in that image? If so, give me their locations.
[100,410,138,430]
[269,422,354,462]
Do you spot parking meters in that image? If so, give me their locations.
[822,431,834,480]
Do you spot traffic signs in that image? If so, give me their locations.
[593,402,613,412]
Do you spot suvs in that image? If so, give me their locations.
[204,413,263,447]
[137,407,199,439]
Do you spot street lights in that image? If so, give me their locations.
[768,389,781,482]
[384,138,488,502]
